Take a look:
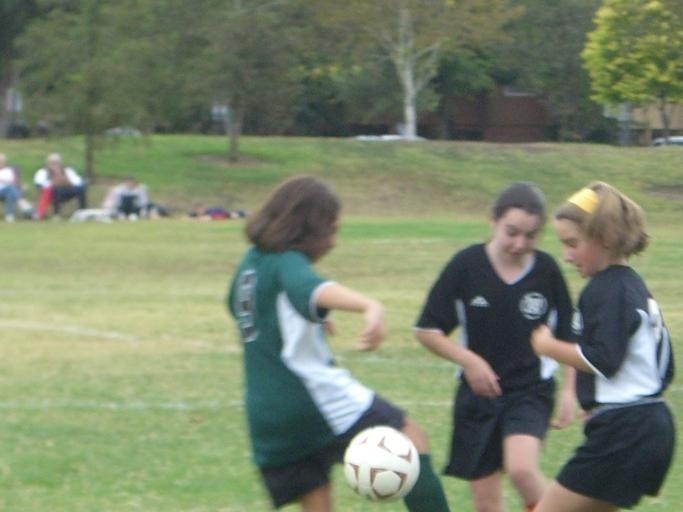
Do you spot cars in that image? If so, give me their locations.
[653,135,682,148]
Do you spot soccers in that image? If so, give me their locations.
[344,425,420,504]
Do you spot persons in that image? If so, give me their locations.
[101,175,149,221]
[226,174,451,512]
[411,180,575,512]
[33,151,89,220]
[0,152,32,222]
[182,204,247,221]
[530,180,677,512]
